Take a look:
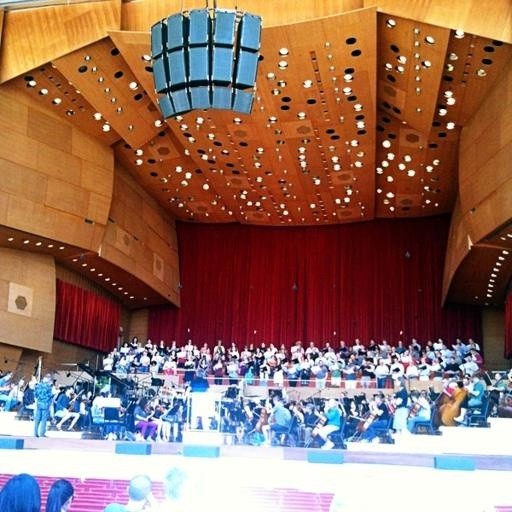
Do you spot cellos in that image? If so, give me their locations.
[255,390,274,432]
[439,387,467,426]
[408,391,425,417]
[311,415,326,438]
[358,405,377,432]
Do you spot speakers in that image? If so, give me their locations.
[150,9,264,120]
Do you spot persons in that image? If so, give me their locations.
[215,401,219,413]
[281,338,483,389]
[391,381,433,435]
[195,416,202,429]
[2,370,190,443]
[220,384,393,448]
[78,358,96,377]
[102,337,288,387]
[208,415,218,430]
[0,463,191,512]
[435,373,486,422]
[488,371,511,406]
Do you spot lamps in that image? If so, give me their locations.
[151,0,262,120]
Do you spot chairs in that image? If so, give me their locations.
[414,393,491,436]
[272,398,397,449]
